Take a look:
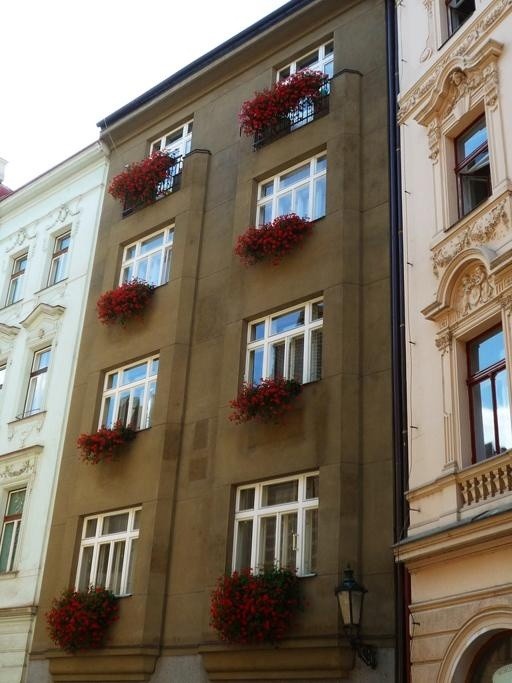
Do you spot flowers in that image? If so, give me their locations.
[231,67,328,138]
[77,411,139,460]
[227,377,305,426]
[209,567,301,643]
[230,211,315,263]
[93,277,154,326]
[48,581,122,654]
[106,148,179,202]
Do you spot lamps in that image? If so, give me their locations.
[335,560,381,670]
